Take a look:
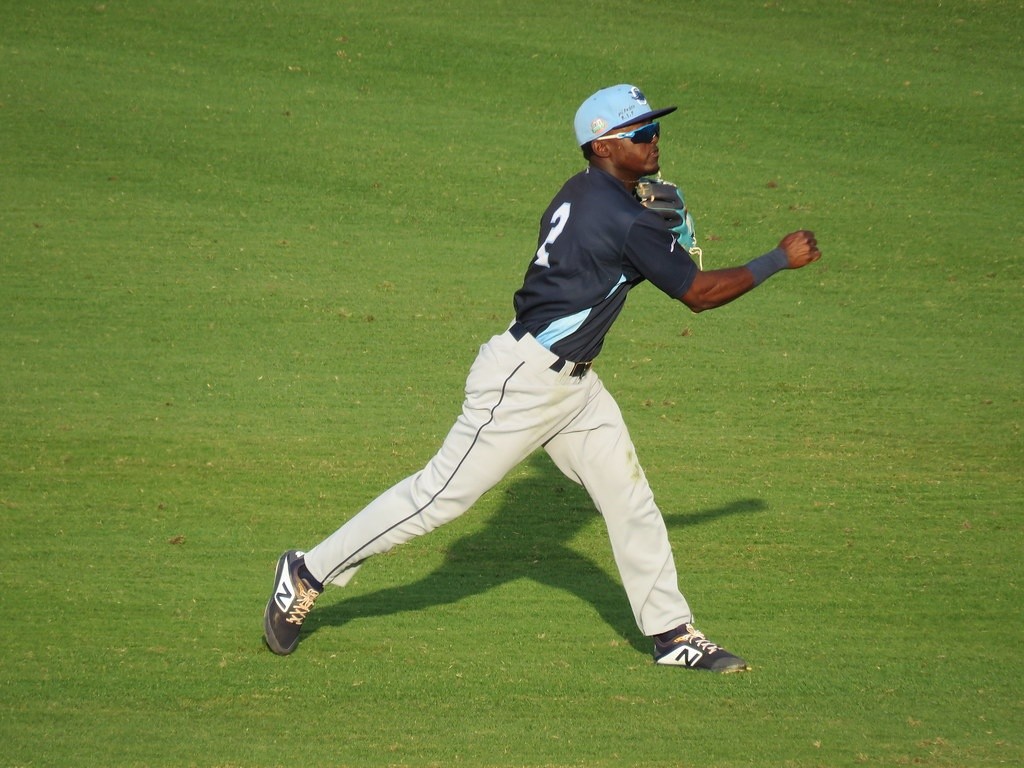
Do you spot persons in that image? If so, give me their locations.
[264,85,820,671]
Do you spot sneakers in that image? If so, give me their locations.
[264,549,324,656]
[653,623,746,673]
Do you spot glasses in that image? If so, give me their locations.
[597,122,661,144]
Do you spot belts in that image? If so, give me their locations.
[509,322,593,379]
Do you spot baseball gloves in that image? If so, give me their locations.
[637,179,696,258]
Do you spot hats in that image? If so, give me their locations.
[573,84,677,146]
